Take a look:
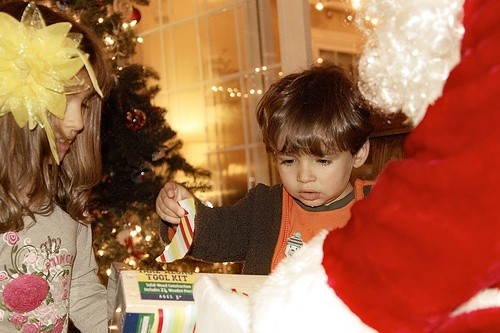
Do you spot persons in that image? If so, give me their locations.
[153,65,372,278]
[0,0,114,332]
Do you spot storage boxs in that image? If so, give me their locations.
[113,269,205,333]
